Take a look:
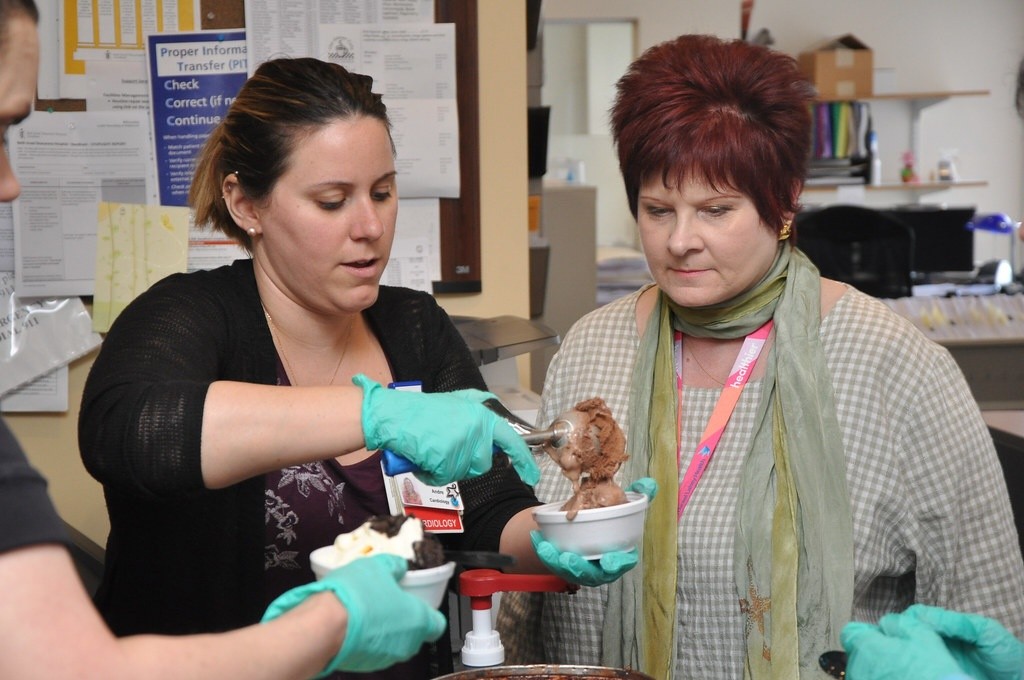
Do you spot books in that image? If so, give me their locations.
[808,95,874,185]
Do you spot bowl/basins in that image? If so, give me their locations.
[310,545,457,610]
[532,493,649,560]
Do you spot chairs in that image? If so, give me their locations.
[797,205,915,296]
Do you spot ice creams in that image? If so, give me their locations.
[329,511,448,577]
[545,396,628,519]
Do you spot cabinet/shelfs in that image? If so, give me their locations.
[804,89,991,192]
[543,179,599,341]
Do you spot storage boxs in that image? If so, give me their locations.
[797,32,875,98]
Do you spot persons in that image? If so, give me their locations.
[73,57,657,680]
[496,35,1024,680]
[0,0,452,680]
[839,604,1024,680]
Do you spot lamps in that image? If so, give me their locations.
[966,212,1016,284]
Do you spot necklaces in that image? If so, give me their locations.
[266,314,352,386]
[686,341,727,386]
